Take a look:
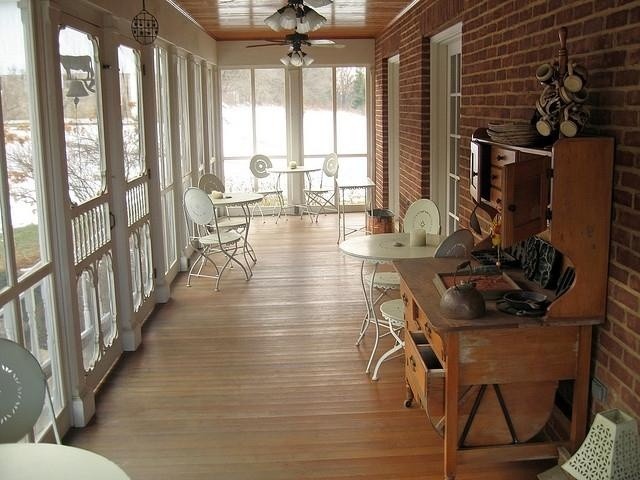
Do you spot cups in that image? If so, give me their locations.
[290,161,297,169]
[409,228,426,248]
[532,63,597,139]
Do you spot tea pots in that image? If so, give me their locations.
[438,259,486,320]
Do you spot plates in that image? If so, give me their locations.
[486,121,536,146]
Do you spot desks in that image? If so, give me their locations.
[338,231,458,375]
[0,433,130,480]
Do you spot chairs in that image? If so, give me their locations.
[371,228,476,382]
[359,197,442,334]
[182,153,377,292]
[0,328,68,448]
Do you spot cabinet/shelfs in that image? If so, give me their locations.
[467,130,615,324]
[393,254,608,480]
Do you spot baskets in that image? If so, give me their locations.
[487,121,533,133]
[485,129,539,147]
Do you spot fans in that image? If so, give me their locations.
[245,33,346,51]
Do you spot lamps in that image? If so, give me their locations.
[262,0,329,39]
[277,45,317,69]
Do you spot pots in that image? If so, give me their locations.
[502,289,548,312]
